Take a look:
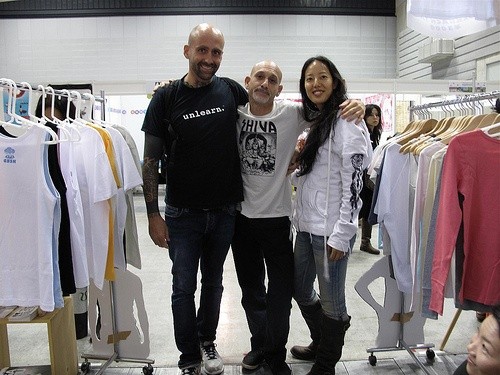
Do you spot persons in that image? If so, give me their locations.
[34,92,102,344]
[141,22,302,375]
[151,61,367,375]
[451,306,500,375]
[290,56,373,375]
[348,104,384,254]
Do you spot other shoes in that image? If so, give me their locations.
[241,347,266,369]
[266,360,293,375]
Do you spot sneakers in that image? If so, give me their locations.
[179,362,201,375]
[200,341,224,374]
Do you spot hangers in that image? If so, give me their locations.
[0,78,114,145]
[382,90,500,156]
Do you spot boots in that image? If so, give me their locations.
[360,218,380,255]
[290,293,321,359]
[305,307,351,375]
